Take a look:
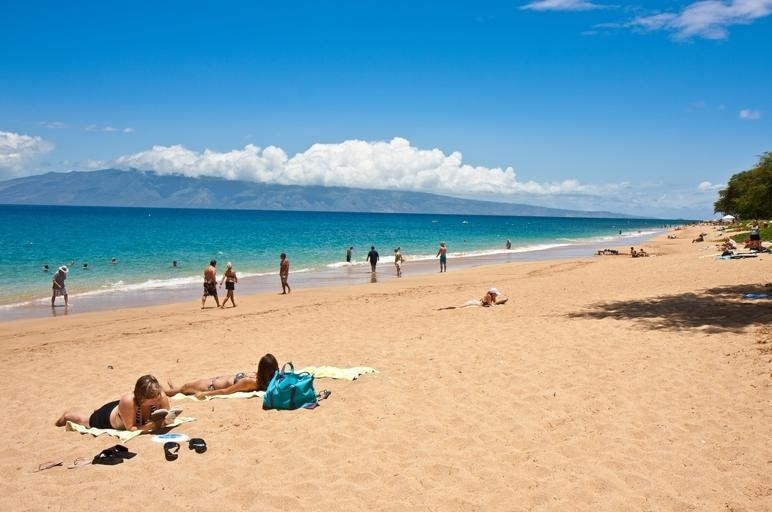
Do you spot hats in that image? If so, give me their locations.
[59,265,68,273]
[488,288,500,295]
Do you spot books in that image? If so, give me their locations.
[146,406,184,430]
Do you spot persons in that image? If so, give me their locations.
[504,240,512,251]
[81,263,91,271]
[161,353,279,399]
[691,234,704,244]
[714,218,764,252]
[50,264,71,308]
[394,246,404,276]
[433,287,508,311]
[200,257,221,310]
[434,240,448,273]
[345,246,354,262]
[366,245,380,272]
[111,256,119,265]
[168,260,182,271]
[631,246,639,257]
[637,248,648,257]
[41,263,52,273]
[278,252,292,295]
[220,261,239,310]
[54,375,173,433]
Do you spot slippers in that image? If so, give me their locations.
[108,444,137,459]
[190,438,207,453]
[164,441,180,461]
[92,449,123,465]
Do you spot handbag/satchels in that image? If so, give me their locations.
[260,361,317,410]
